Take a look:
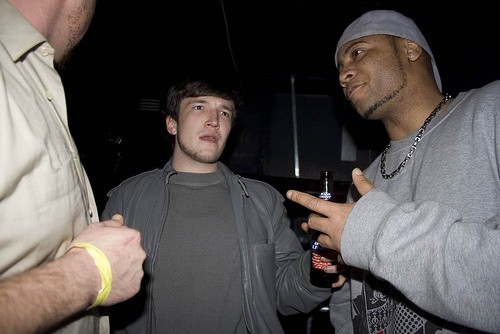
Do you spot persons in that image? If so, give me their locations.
[285,10,500,333]
[99,78,351,334]
[0,0,147,334]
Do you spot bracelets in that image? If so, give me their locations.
[65,241,112,311]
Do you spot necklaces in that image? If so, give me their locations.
[379,93,450,180]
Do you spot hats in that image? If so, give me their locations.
[335,11,442,97]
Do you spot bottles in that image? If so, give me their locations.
[309,171,347,289]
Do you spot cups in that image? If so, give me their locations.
[295,216,313,244]
